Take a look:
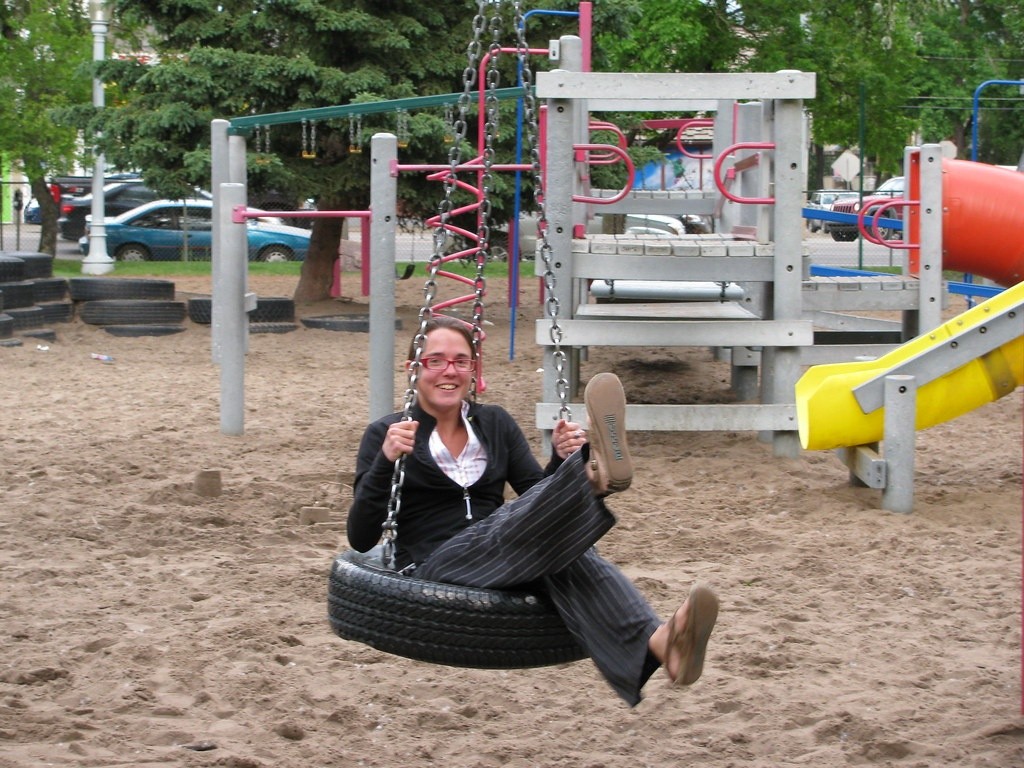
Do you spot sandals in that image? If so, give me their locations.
[663,582,720,686]
[584,373,633,493]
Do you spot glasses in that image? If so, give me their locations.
[410,356,477,373]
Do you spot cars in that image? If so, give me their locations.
[24,194,77,225]
[50,174,282,239]
[807,173,910,243]
[78,197,316,263]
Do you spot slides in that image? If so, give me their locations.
[794,277,1024,450]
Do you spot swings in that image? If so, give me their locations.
[327,0,588,671]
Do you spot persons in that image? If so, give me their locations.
[671,158,685,184]
[345,317,720,710]
